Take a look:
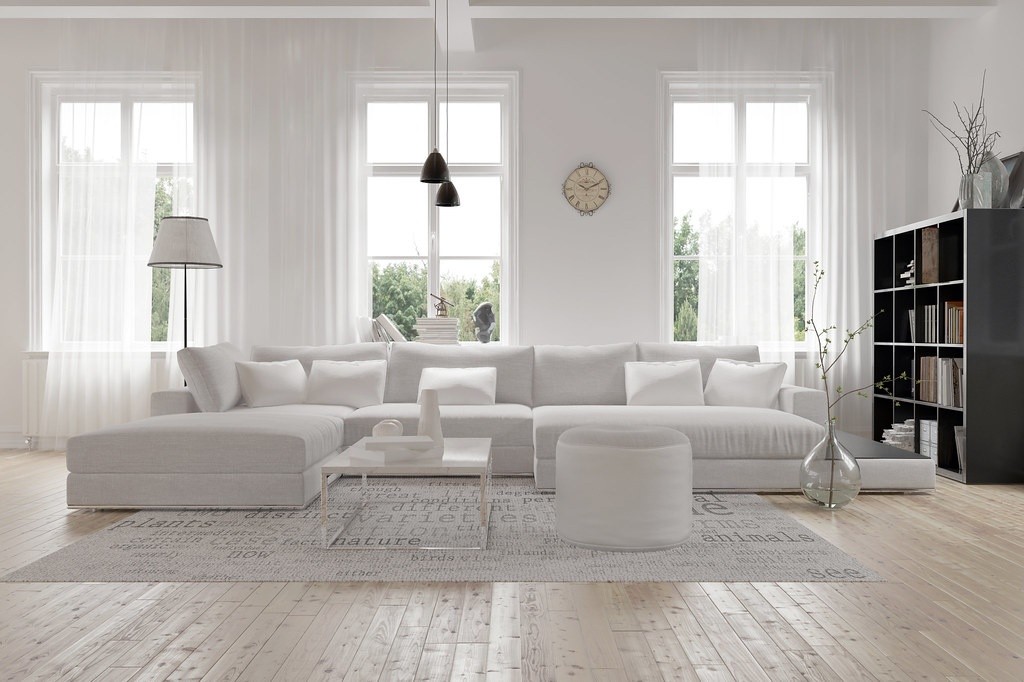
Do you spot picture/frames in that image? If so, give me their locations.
[951,151,1024,213]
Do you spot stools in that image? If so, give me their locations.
[556,424,693,553]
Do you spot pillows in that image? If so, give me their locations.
[234,358,308,408]
[417,367,497,405]
[304,360,387,408]
[177,342,242,412]
[703,359,787,409]
[624,359,705,407]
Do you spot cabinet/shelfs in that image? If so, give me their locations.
[872,209,1024,485]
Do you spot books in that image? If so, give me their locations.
[920,357,937,403]
[924,305,936,343]
[908,309,915,343]
[944,302,963,344]
[413,318,460,346]
[938,357,963,408]
[370,314,406,342]
[912,360,914,396]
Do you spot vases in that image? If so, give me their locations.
[976,151,1010,209]
[960,172,992,209]
[799,421,862,508]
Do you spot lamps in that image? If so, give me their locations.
[435,1,460,207]
[420,1,449,183]
[146,216,223,388]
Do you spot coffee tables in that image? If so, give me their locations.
[320,436,493,550]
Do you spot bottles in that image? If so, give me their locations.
[419,388,444,459]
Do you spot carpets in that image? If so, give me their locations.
[0,476,887,583]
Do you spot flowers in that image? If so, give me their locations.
[804,261,939,508]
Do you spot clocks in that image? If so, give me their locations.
[561,162,611,216]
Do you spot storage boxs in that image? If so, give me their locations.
[920,420,937,465]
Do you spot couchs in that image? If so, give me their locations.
[65,341,935,509]
